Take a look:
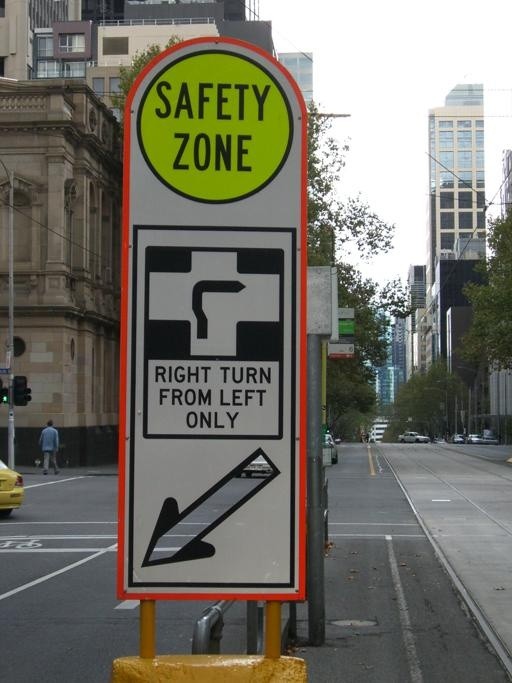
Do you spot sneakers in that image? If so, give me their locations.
[43,470,61,475]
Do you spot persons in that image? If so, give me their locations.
[38,419,61,475]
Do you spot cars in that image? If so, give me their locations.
[0,459,24,518]
[325,434,341,464]
[452,434,499,444]
[236,454,274,478]
[397,432,430,443]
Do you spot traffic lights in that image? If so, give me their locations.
[0,375,32,407]
